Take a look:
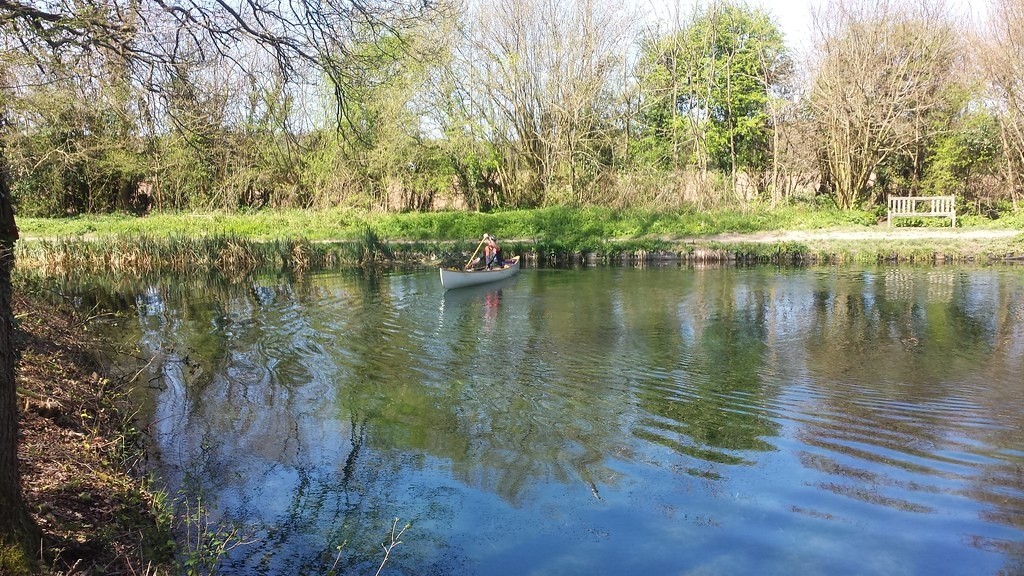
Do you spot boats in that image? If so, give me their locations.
[439,255,520,289]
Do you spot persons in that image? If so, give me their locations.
[470,233,504,271]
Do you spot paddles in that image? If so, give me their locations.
[463,236,486,268]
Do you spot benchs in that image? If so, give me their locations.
[888,193,955,228]
